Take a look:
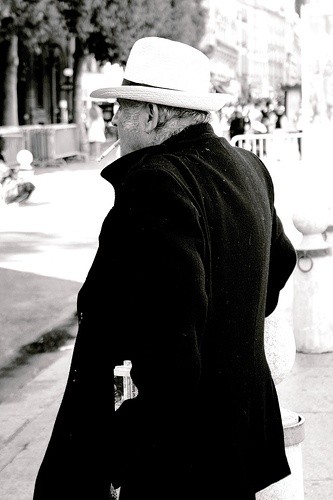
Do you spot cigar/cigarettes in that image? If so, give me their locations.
[97,138,120,163]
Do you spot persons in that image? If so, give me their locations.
[87,96,287,160]
[32,36,297,500]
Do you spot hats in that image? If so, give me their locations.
[88,36,234,113]
